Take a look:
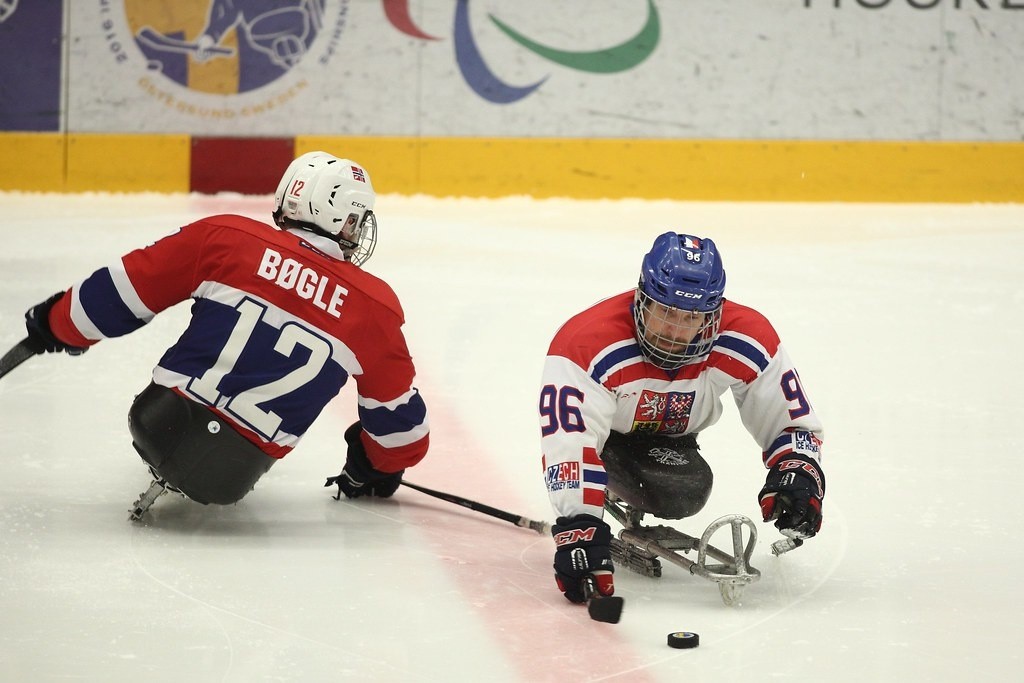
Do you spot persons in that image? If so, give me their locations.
[23,152,430,506]
[537,232,826,604]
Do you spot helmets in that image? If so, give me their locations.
[272,151,377,267]
[634,231,726,370]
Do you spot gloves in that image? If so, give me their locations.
[758,452,825,540]
[551,514,615,604]
[324,421,405,501]
[25,292,89,356]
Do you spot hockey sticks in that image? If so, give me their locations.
[1,336,36,377]
[769,495,804,556]
[583,575,626,623]
[323,471,552,538]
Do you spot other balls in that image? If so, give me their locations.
[667,631,699,650]
